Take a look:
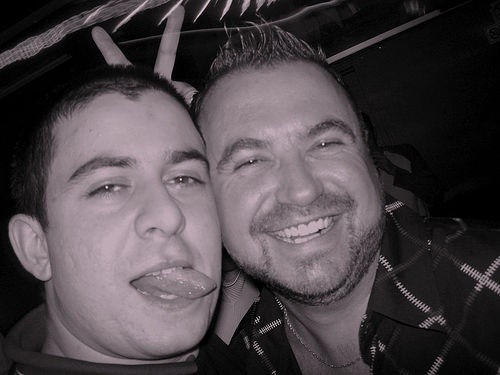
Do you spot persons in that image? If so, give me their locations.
[189,13,500,375]
[197,238,266,375]
[1,65,224,374]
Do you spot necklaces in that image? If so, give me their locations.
[221,270,242,288]
[274,294,365,368]
